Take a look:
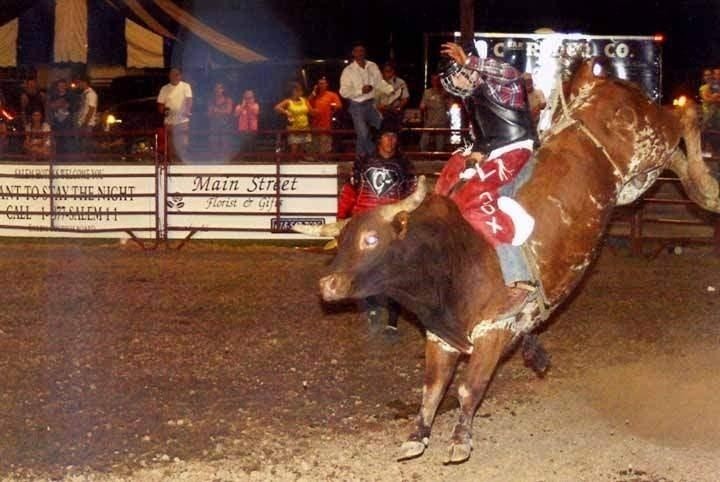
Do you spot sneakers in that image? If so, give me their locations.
[365,309,400,338]
[493,282,539,321]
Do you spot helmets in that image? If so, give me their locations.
[437,42,481,78]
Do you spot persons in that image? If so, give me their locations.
[698,67,720,155]
[1,59,98,162]
[158,67,343,162]
[336,120,420,333]
[376,61,450,163]
[520,72,549,133]
[441,93,465,161]
[433,35,544,323]
[705,66,720,108]
[339,38,396,157]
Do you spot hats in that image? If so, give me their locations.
[370,116,404,144]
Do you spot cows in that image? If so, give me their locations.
[287,54,720,464]
